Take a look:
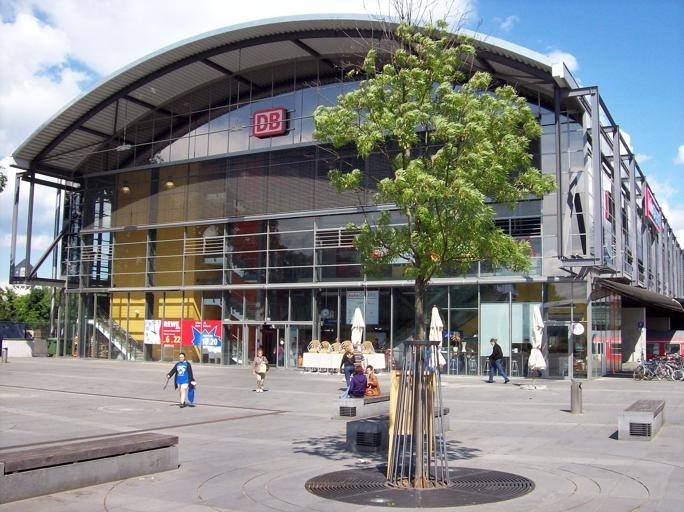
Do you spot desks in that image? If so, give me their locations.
[302,354,385,373]
[375,349,403,372]
[439,351,461,374]
[460,352,477,375]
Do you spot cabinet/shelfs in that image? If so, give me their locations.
[574,322,587,375]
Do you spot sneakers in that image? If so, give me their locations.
[180,401,186,408]
[256,388,264,393]
[486,379,510,384]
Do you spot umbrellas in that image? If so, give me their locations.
[425,302,447,372]
[349,305,366,345]
[527,303,546,384]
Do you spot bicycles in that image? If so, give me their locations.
[633,352,684,383]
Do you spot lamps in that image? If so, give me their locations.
[120,180,132,195]
[116,102,132,152]
[162,176,175,188]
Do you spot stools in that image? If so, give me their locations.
[450,359,520,376]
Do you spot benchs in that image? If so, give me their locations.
[346,407,449,453]
[618,400,664,441]
[0,433,180,505]
[333,393,390,419]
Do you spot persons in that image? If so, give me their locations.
[339,342,355,388]
[485,337,509,384]
[251,348,268,394]
[352,342,365,376]
[272,338,284,367]
[338,365,367,399]
[364,366,382,397]
[164,352,198,408]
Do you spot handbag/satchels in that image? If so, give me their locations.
[187,385,195,402]
[257,357,270,372]
